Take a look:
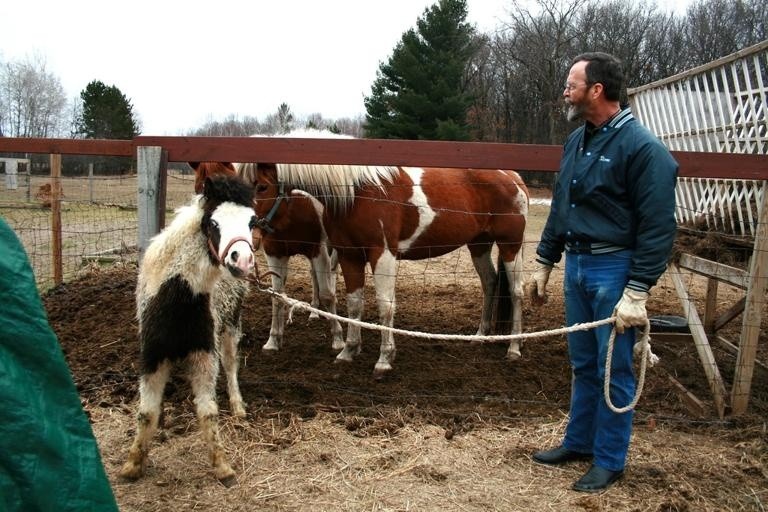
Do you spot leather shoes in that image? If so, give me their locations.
[572,462,624,492]
[532,444,594,464]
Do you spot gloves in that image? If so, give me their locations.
[525,260,552,309]
[610,286,651,335]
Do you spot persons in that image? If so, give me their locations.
[524,52,680,495]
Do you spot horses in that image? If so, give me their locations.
[235,127,531,382]
[116,174,259,490]
[187,161,347,357]
